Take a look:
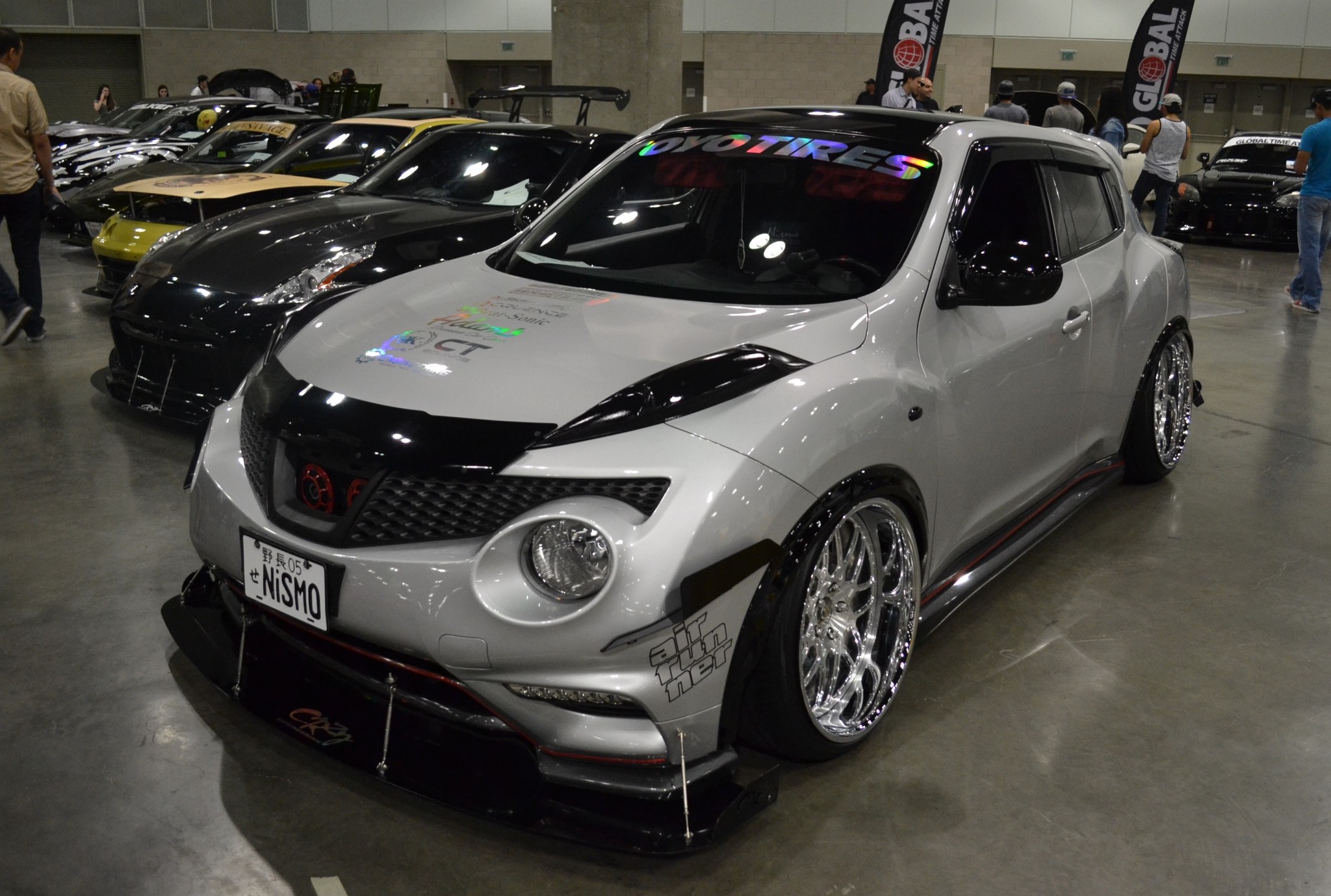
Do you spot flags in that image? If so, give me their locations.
[874,0,950,105]
[1121,0,1195,132]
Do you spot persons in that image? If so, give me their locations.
[158,85,169,98]
[1132,94,1190,240]
[983,80,1029,126]
[342,68,358,84]
[855,68,938,109]
[311,78,323,93]
[1285,88,1331,313]
[285,79,303,106]
[190,74,208,96]
[328,70,342,85]
[94,85,116,116]
[300,80,318,101]
[1041,82,1084,134]
[0,26,64,348]
[1088,87,1128,156]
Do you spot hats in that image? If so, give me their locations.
[1057,81,1076,99]
[864,79,876,84]
[1306,90,1331,110]
[1159,93,1182,107]
[998,81,1014,95]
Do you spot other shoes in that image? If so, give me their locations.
[0,303,33,346]
[27,328,47,342]
[1292,299,1321,312]
[1285,285,1300,302]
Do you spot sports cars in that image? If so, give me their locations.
[1165,130,1308,247]
[44,67,700,436]
[160,103,1205,859]
